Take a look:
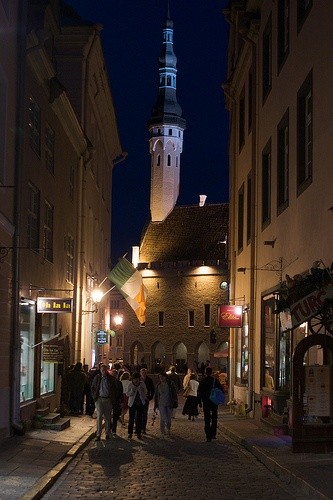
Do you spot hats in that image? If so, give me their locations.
[131,372,141,379]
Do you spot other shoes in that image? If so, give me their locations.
[95,436,100,441]
[128,434,132,439]
[188,417,195,421]
[137,434,141,439]
[160,433,164,438]
[106,435,112,439]
[166,432,170,437]
[207,436,216,443]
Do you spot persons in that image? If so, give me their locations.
[192,360,212,391]
[108,369,123,437]
[135,368,154,434]
[62,360,227,421]
[182,374,200,422]
[265,369,274,389]
[68,364,76,375]
[91,364,116,441]
[154,372,179,433]
[182,368,196,390]
[197,366,223,443]
[126,373,148,438]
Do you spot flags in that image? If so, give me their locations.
[116,279,149,325]
[106,255,146,310]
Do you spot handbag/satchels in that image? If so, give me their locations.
[183,380,191,397]
[168,384,178,408]
[209,377,225,406]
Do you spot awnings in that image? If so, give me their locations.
[213,349,228,357]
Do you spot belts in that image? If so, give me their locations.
[99,396,112,398]
